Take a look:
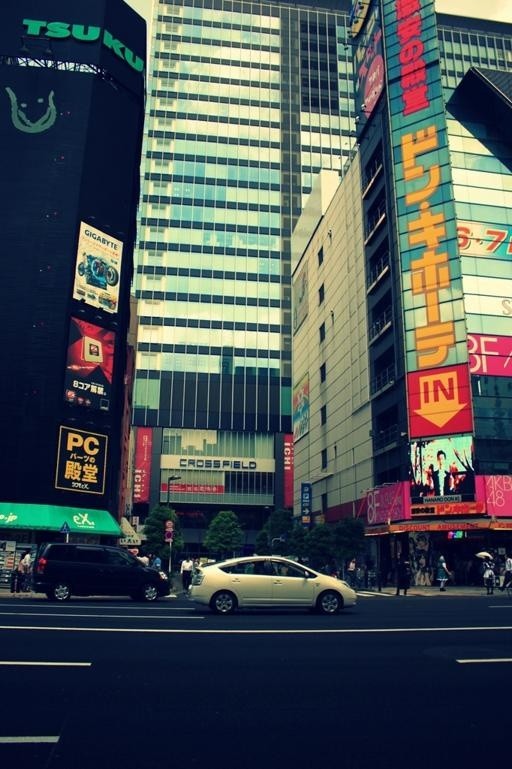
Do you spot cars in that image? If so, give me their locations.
[187,554,357,614]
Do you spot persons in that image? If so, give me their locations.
[428,450,453,496]
[178,555,199,596]
[435,555,452,591]
[482,558,496,595]
[135,551,163,571]
[409,533,433,586]
[497,552,512,592]
[396,552,409,596]
[16,548,36,593]
[347,557,356,587]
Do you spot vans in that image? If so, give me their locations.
[30,542,172,604]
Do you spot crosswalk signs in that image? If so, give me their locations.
[59,522,72,534]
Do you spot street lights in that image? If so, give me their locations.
[167,476,182,507]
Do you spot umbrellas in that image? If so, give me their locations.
[473,550,493,561]
